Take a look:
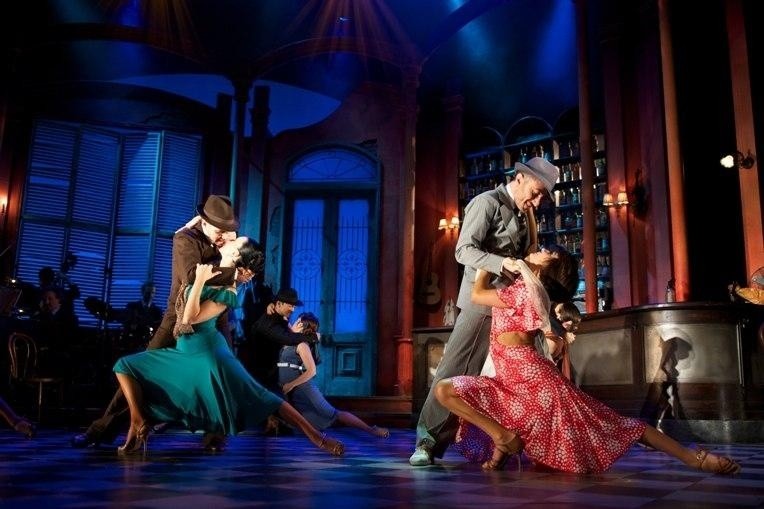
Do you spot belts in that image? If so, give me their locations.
[277,362,303,371]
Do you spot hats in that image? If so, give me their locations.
[194,194,241,233]
[272,285,304,308]
[514,156,560,204]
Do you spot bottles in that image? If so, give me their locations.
[465,155,503,202]
[519,137,608,280]
[665,282,676,303]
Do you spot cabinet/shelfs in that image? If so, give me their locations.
[409,296,764,427]
[453,101,617,323]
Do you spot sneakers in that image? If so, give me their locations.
[8,415,36,437]
[69,430,102,449]
[368,424,391,441]
[201,432,226,451]
[409,442,436,466]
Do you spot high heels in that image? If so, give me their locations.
[117,421,148,455]
[480,434,526,473]
[261,415,281,438]
[692,444,742,477]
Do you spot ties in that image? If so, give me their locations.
[212,242,219,256]
[512,206,529,256]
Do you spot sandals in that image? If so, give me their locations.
[316,431,346,457]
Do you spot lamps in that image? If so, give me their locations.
[603,190,630,220]
[719,146,754,168]
[437,215,462,238]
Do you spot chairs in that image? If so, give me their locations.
[5,329,67,426]
